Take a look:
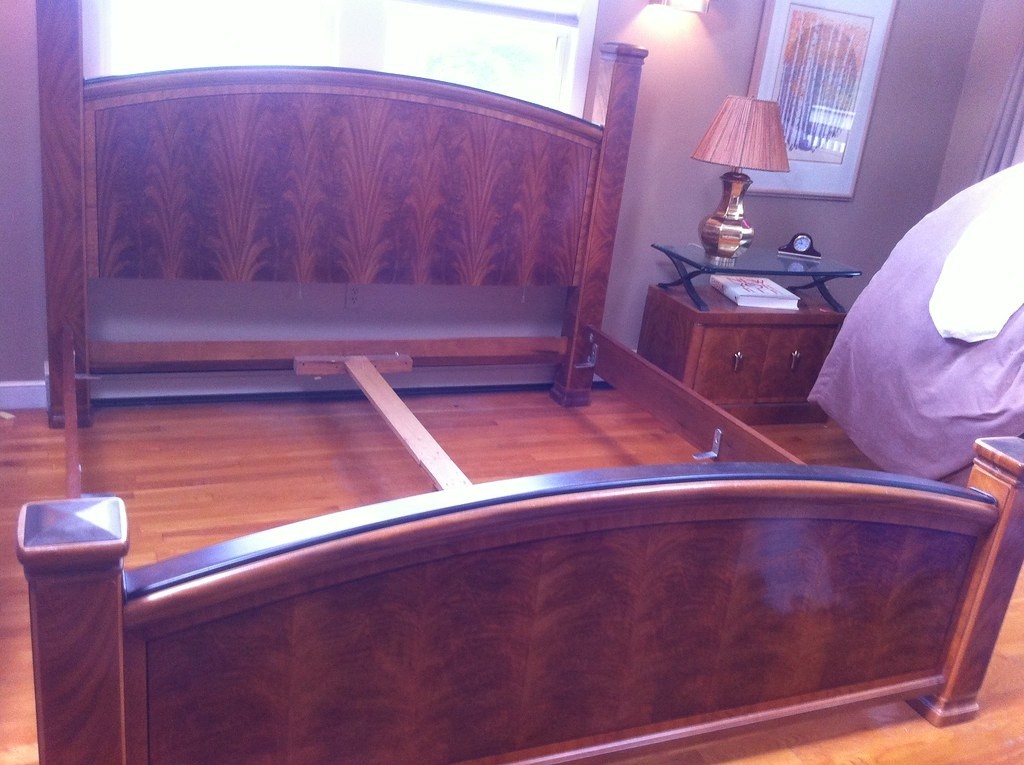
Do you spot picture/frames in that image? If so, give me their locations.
[736,0,900,204]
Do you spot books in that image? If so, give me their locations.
[710,275,800,310]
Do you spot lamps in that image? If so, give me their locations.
[649,0,710,14]
[690,94,790,257]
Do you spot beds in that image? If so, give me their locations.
[13,0,1024,765]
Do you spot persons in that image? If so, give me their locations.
[808,163,1024,480]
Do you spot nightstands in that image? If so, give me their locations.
[636,243,863,422]
